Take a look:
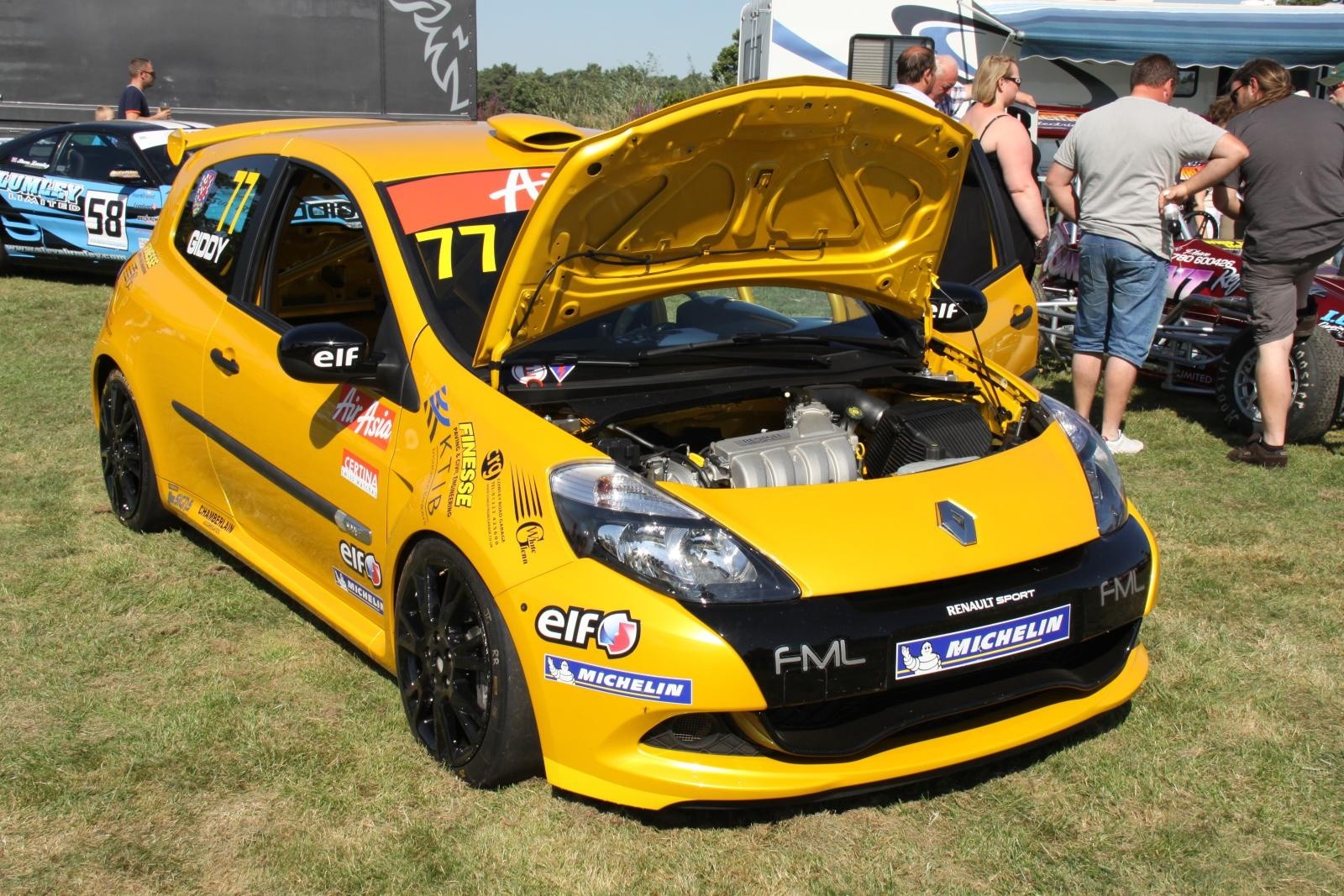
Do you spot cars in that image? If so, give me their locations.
[93,74,1161,811]
[0,120,363,278]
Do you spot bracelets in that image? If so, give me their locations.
[1034,239,1049,248]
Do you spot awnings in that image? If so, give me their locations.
[973,0,1344,70]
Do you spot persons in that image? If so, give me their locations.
[890,45,940,110]
[1193,62,1344,239]
[118,58,171,121]
[960,54,1049,285]
[1045,52,1249,455]
[92,106,118,146]
[1213,58,1344,469]
[926,53,959,106]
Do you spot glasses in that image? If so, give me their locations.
[1230,84,1248,104]
[1329,83,1344,91]
[1002,75,1021,87]
[136,70,155,78]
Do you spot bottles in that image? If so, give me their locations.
[1162,189,1181,234]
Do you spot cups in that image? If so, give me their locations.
[160,100,170,111]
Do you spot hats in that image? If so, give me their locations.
[1314,63,1344,87]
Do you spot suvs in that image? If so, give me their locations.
[1029,101,1344,446]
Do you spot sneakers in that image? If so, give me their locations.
[1227,441,1289,469]
[1101,427,1144,455]
